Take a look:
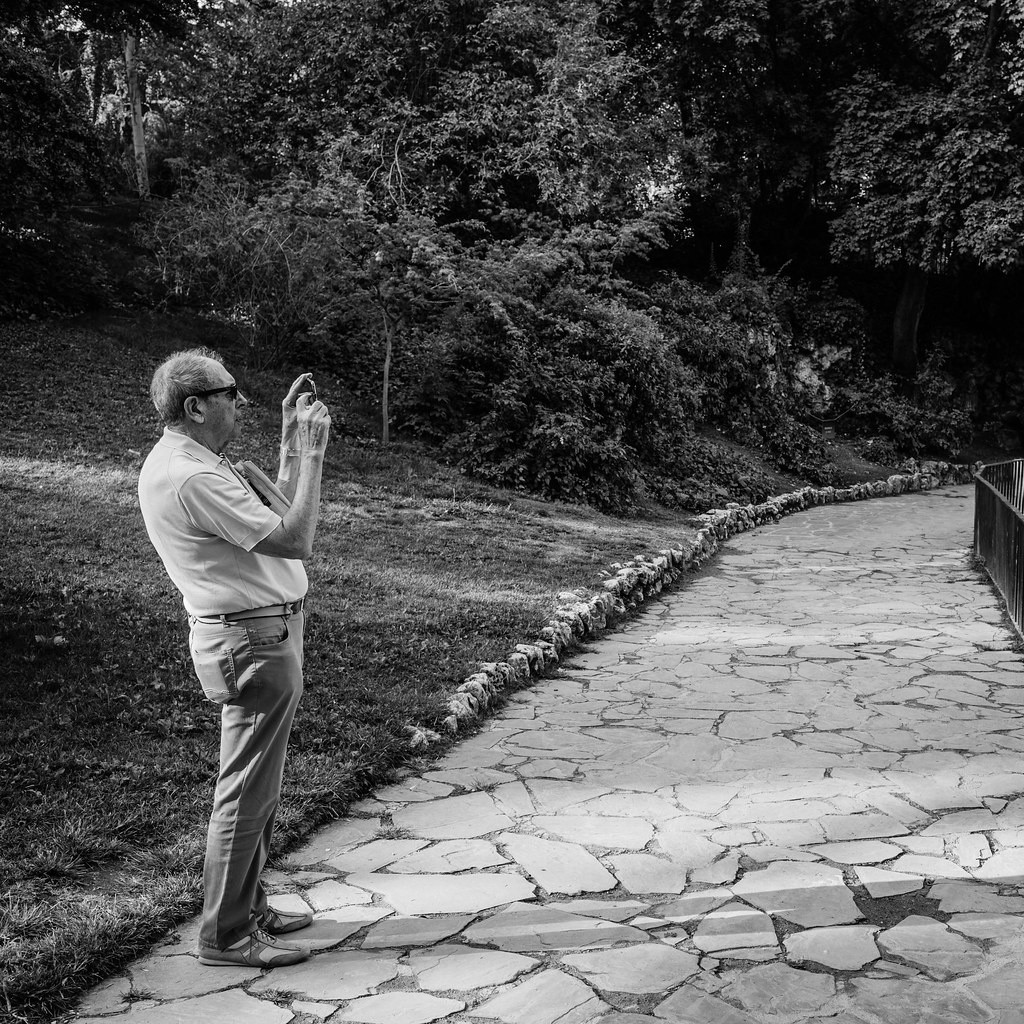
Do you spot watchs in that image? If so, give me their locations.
[278,445,302,457]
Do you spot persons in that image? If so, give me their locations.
[137,348,332,967]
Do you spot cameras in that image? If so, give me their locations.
[297,377,318,406]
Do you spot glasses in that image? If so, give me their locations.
[180,383,238,411]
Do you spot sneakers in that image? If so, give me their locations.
[257,905,313,935]
[198,928,310,967]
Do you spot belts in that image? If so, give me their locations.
[195,597,305,624]
